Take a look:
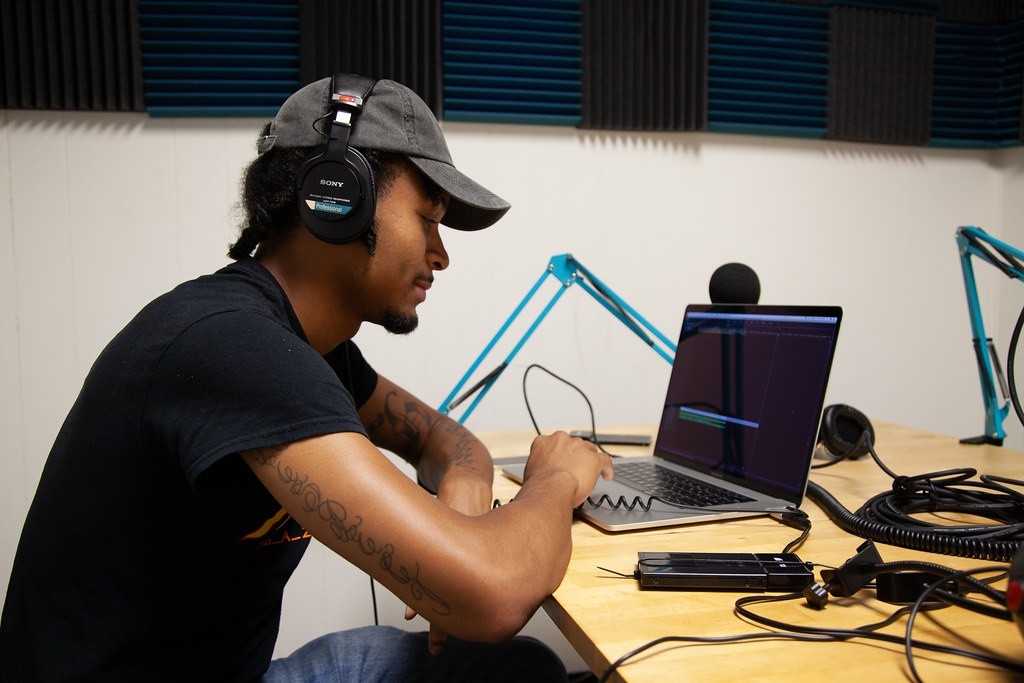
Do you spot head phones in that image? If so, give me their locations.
[817,404,875,460]
[295,73,381,245]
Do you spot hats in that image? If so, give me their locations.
[257,76,512,231]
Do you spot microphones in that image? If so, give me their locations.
[706,262,761,306]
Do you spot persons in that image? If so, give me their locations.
[0,73,616,683]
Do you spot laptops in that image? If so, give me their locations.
[503,304,845,531]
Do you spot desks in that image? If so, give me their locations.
[477,428,1024,682]
